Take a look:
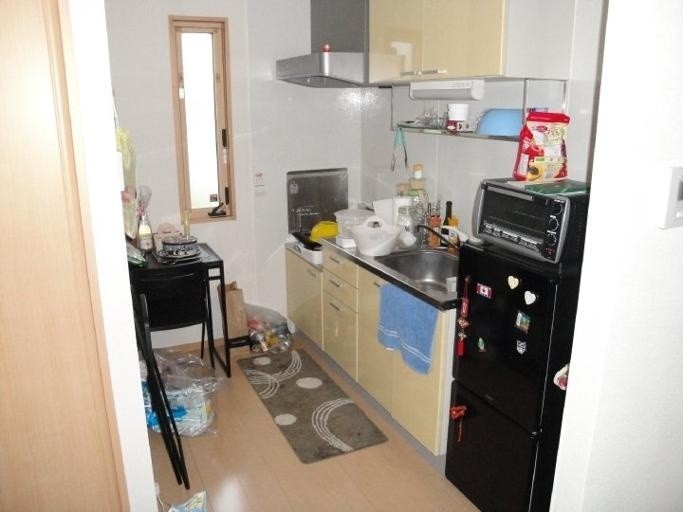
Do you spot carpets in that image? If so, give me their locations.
[236,346,389,465]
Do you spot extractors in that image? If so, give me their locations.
[273,0,369,89]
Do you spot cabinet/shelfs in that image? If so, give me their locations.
[367,1,575,86]
[321,243,358,387]
[283,246,322,350]
[358,263,457,457]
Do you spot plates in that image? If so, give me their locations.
[156,248,203,262]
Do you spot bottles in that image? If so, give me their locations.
[448,216,460,246]
[397,179,429,252]
[446,102,468,134]
[439,201,454,245]
[414,163,422,179]
[426,215,439,246]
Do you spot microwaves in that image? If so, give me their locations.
[471,177,589,268]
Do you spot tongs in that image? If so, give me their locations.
[389,126,408,171]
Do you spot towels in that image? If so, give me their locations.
[375,280,436,379]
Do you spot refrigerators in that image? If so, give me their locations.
[444,239,581,511]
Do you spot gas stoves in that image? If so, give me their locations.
[291,231,322,248]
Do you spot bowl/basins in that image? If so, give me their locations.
[351,217,402,256]
[310,220,338,244]
[474,109,522,137]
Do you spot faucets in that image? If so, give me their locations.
[416,224,459,257]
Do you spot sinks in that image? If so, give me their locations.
[374,249,459,296]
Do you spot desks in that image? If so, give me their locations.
[125,241,232,379]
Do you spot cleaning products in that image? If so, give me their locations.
[448,216,459,253]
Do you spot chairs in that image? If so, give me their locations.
[133,260,215,382]
